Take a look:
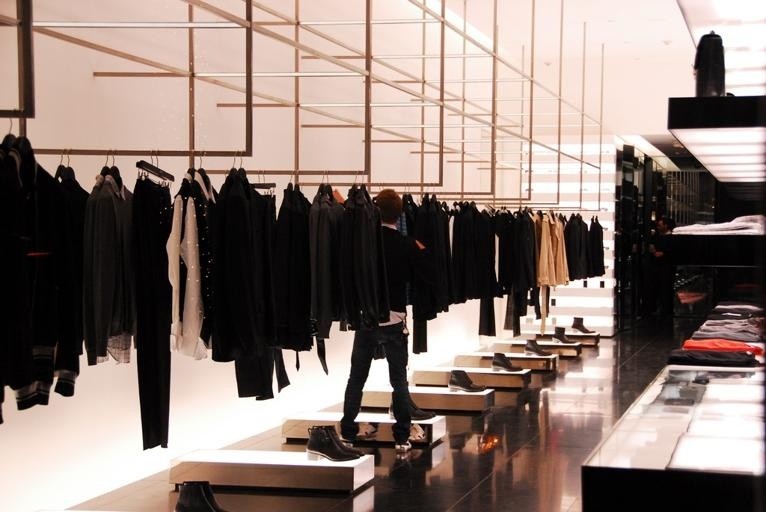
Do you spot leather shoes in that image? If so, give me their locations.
[395,441,413,452]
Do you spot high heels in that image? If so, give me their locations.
[306,428,361,462]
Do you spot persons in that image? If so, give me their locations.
[635,217,675,322]
[336,189,442,455]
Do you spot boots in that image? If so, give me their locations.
[183,481,228,512]
[448,369,488,394]
[524,340,553,356]
[491,352,523,371]
[571,317,596,334]
[312,425,366,457]
[388,390,437,421]
[175,484,214,512]
[552,326,577,344]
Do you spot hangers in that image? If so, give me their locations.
[1,108,271,203]
[282,168,446,226]
[456,199,600,229]
